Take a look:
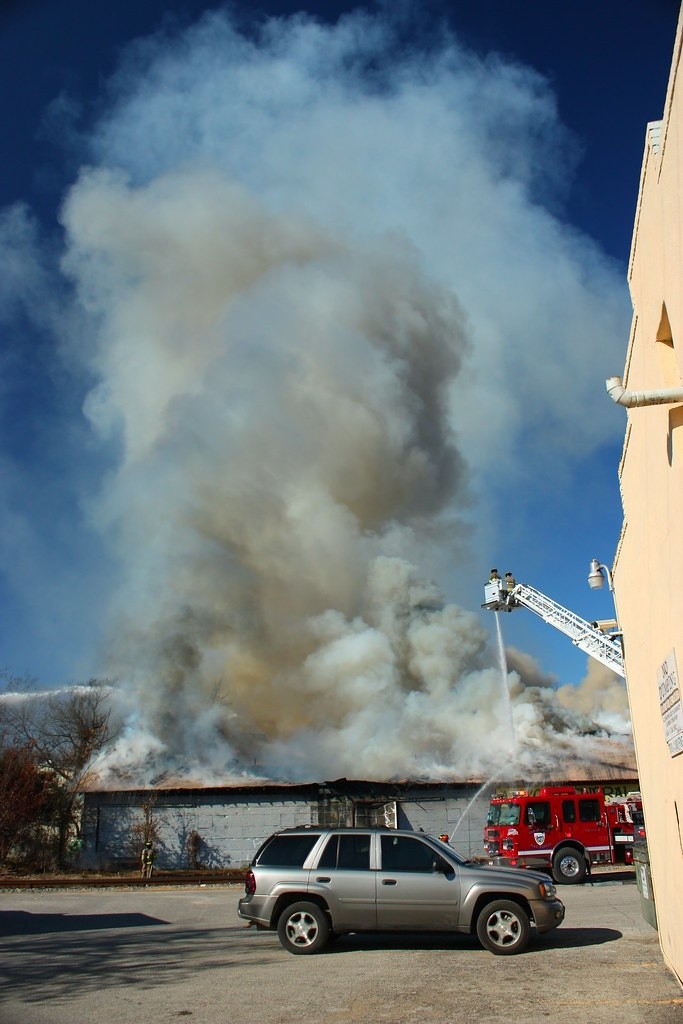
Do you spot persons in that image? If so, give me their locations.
[505,571,515,594]
[489,568,501,585]
[141,842,153,878]
[439,834,449,843]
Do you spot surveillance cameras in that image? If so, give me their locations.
[590,618,616,631]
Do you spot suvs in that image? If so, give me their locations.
[237,824,566,956]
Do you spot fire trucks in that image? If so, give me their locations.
[483,581,645,885]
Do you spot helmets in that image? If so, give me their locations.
[146,842,152,846]
[440,836,448,841]
